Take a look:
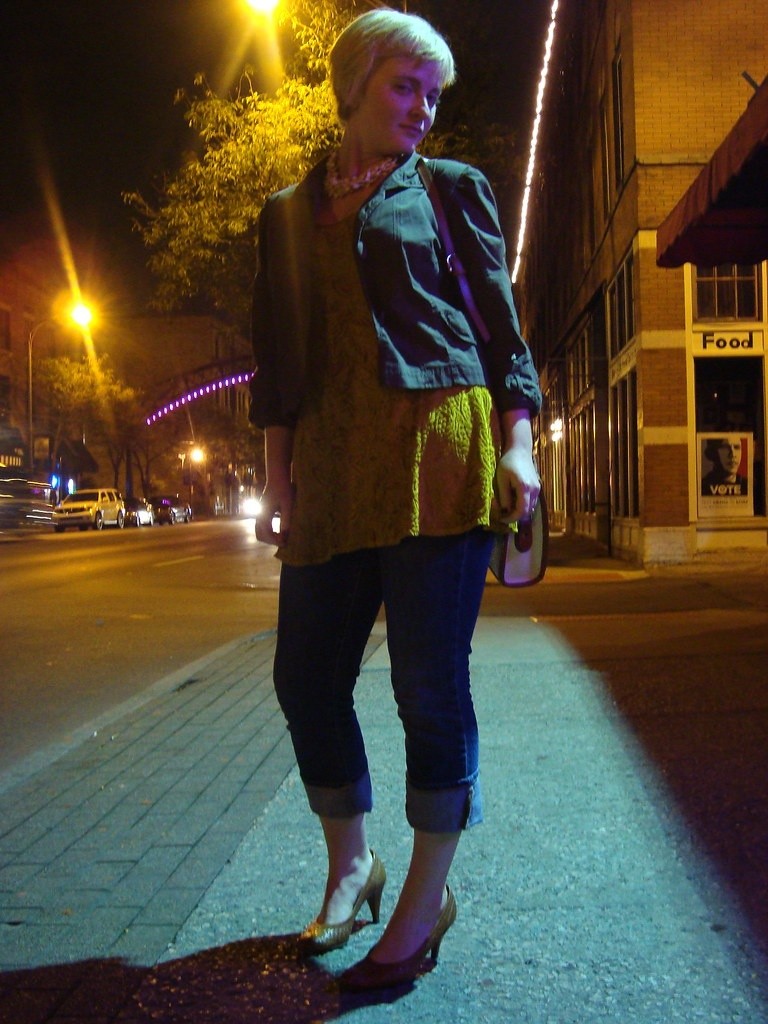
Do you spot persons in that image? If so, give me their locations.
[701,437,748,497]
[248,9,542,987]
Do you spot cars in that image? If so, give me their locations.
[148,496,194,523]
[50,488,127,530]
[123,496,155,526]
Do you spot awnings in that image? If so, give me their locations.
[656,75,767,268]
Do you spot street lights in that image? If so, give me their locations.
[187,448,203,519]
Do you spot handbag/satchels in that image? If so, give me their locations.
[488,473,548,587]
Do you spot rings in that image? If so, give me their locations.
[530,508,535,512]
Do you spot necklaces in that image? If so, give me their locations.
[324,148,403,199]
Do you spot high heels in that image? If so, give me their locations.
[295,849,387,951]
[338,885,457,991]
